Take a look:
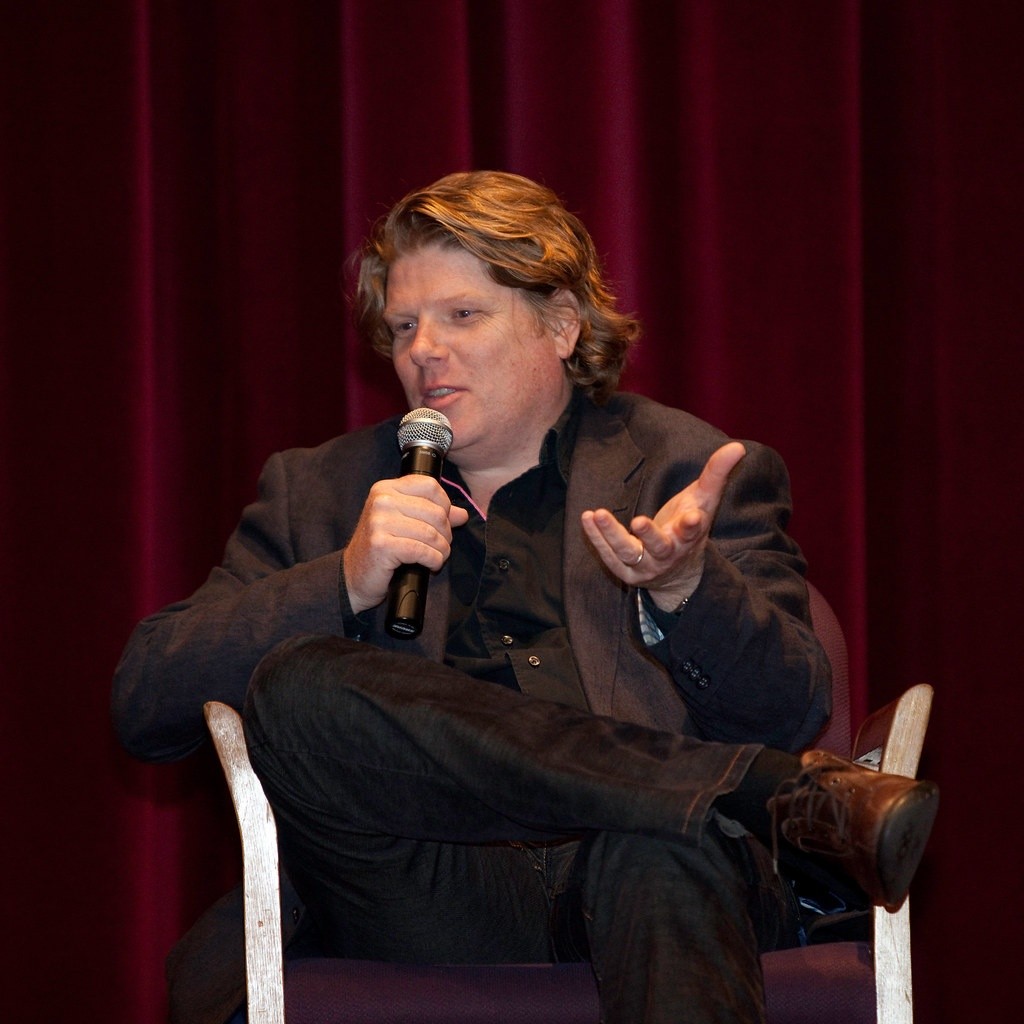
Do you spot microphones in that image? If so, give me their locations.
[384,407,454,640]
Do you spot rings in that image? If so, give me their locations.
[631,545,644,565]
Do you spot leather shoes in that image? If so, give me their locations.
[772,752,939,914]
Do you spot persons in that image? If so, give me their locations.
[110,170,941,1024]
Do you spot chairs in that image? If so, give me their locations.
[201,578,937,1024]
